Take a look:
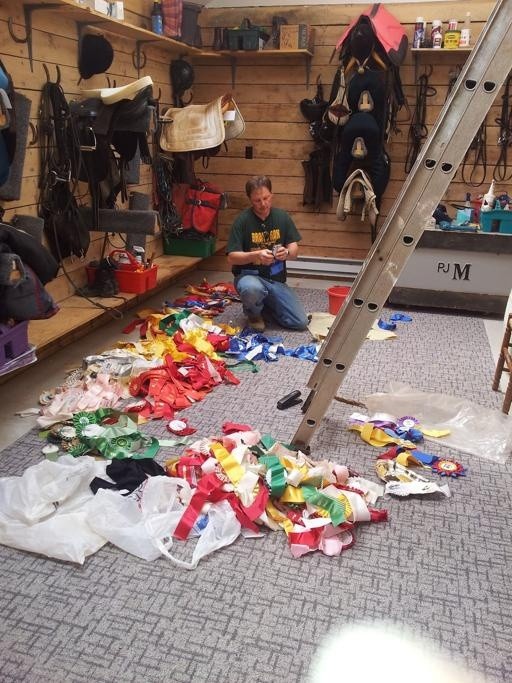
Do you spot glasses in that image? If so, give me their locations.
[260,223,269,239]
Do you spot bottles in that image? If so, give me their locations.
[460,9,472,48]
[444,30,460,49]
[151,0,164,36]
[413,16,425,48]
[448,16,457,30]
[432,31,442,48]
[430,18,443,46]
[481,178,495,210]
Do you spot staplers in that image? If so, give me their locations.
[277,390,302,410]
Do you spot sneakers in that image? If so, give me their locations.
[247,314,265,333]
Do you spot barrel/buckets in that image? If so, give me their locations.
[326,287,351,314]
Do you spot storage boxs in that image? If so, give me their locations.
[162,235,217,257]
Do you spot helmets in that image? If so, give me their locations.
[79,34,113,80]
[301,98,329,122]
[171,59,194,90]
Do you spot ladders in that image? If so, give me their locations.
[288,0,511,455]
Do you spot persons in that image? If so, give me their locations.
[224,173,311,332]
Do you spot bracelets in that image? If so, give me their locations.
[287,249,290,256]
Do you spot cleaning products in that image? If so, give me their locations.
[481,179,497,211]
